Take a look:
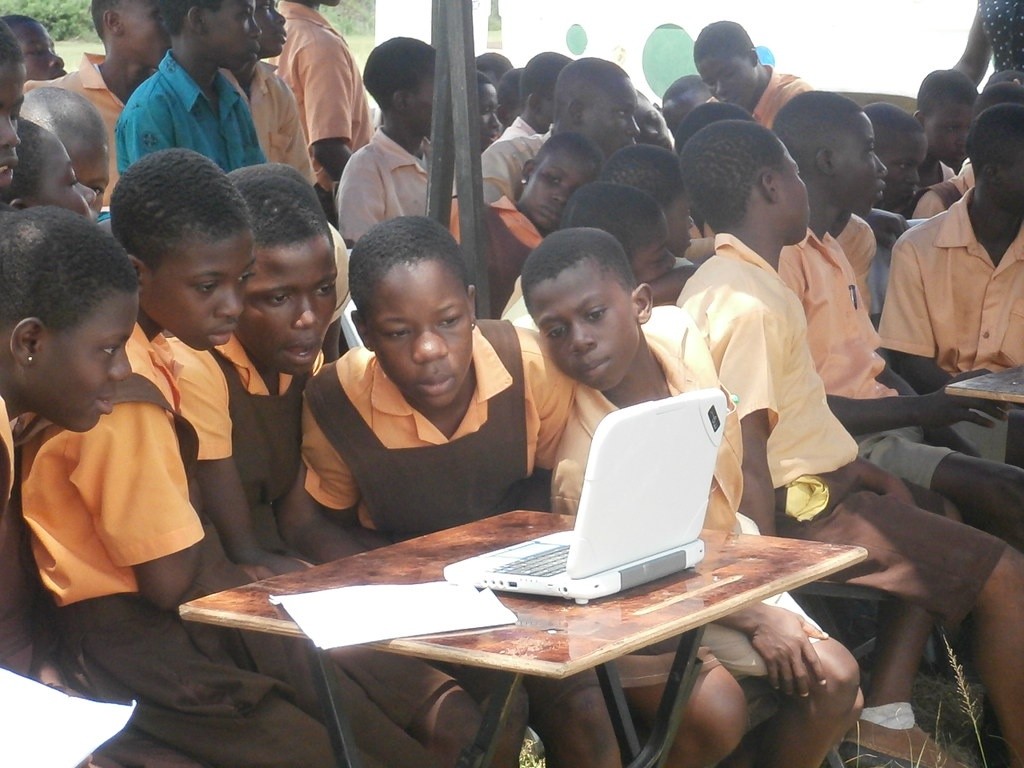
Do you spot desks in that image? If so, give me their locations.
[944,365,1024,468]
[178,511,870,768]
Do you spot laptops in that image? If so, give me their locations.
[444,388,728,604]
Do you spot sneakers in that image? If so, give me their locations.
[839,718,960,768]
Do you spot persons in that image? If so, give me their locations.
[216,0,338,219]
[0,13,485,768]
[448,20,814,322]
[950,0,1023,87]
[22,0,173,206]
[115,1,267,177]
[336,36,437,250]
[260,1,374,226]
[298,216,865,767]
[605,69,1024,768]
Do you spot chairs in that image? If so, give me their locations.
[740,677,846,768]
[791,578,895,664]
